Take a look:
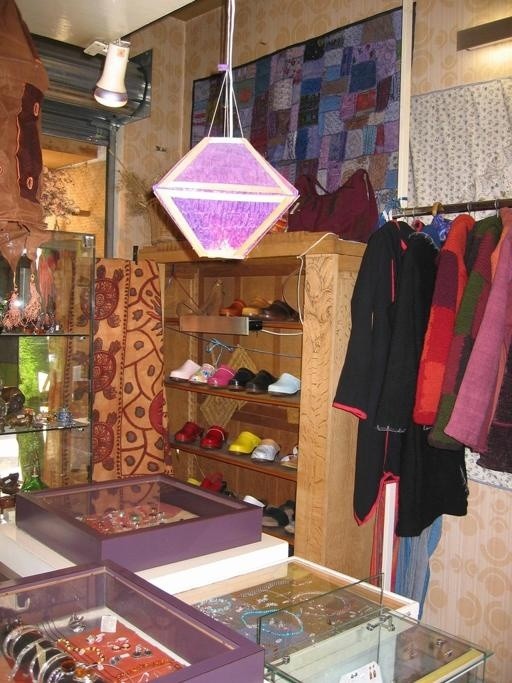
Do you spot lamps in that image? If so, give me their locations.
[83,39,132,109]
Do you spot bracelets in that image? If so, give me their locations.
[2,624,81,683]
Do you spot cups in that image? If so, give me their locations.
[57,410,73,422]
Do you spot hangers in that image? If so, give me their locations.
[381,196,512,228]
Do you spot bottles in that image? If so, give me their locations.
[23,466,49,490]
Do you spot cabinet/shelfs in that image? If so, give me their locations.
[171,558,494,683]
[1,229,97,525]
[139,232,387,582]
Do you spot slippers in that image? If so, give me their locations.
[187,473,294,533]
[176,422,297,469]
[221,298,300,322]
[169,360,300,395]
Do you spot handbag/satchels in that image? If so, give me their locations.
[288,172,374,241]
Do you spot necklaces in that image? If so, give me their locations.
[196,579,347,636]
[55,638,104,670]
